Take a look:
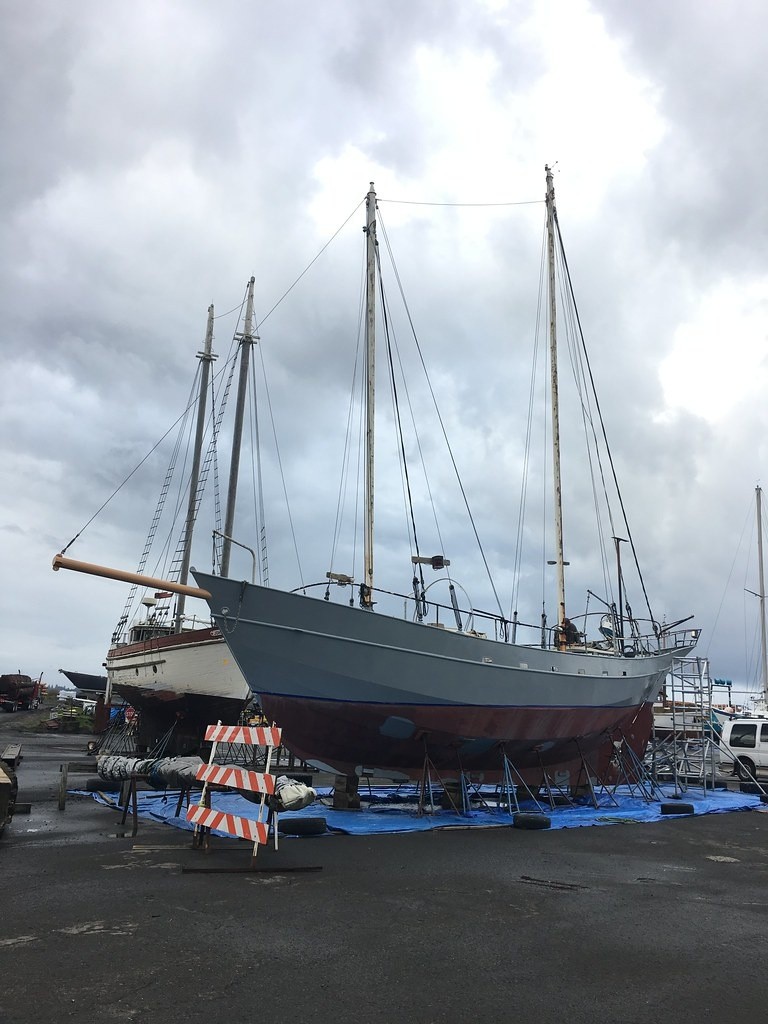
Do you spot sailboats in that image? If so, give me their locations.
[651,614,723,739]
[50,161,706,792]
[689,485,768,737]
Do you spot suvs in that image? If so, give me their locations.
[719,713,768,782]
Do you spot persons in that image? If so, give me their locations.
[561,617,581,643]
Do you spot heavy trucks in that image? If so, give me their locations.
[0,671,47,712]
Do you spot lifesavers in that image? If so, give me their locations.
[278,817,327,836]
[760,793,768,802]
[514,812,551,830]
[660,803,694,814]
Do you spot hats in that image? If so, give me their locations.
[561,618,570,624]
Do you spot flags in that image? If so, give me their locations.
[155,591,174,598]
